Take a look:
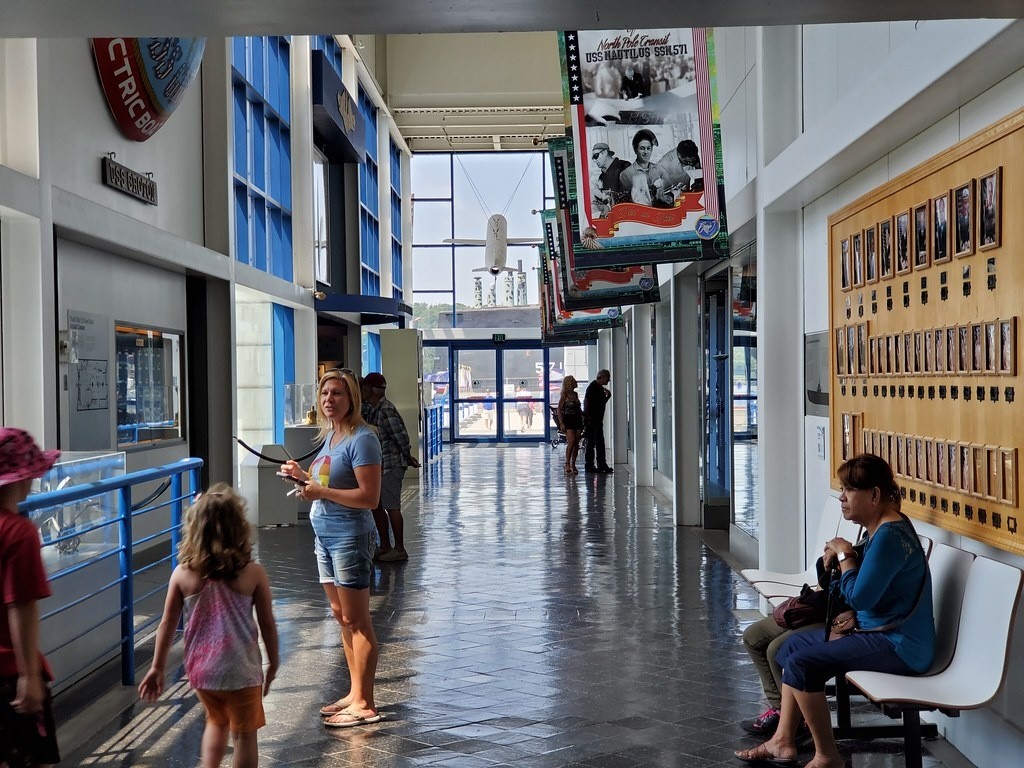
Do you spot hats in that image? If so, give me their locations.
[593,143,615,156]
[364,372,387,388]
[0,427,61,485]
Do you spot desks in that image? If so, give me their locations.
[134,426,179,443]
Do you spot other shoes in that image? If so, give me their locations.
[597,464,613,473]
[584,464,598,472]
[373,546,392,558]
[379,547,409,561]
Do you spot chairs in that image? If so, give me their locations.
[739,512,1024,768]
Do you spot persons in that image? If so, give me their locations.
[136,481,278,768]
[515,386,535,433]
[481,389,496,429]
[584,369,614,473]
[280,369,383,727]
[0,428,62,768]
[732,451,937,768]
[582,53,696,102]
[557,374,584,476]
[359,372,422,561]
[590,129,701,223]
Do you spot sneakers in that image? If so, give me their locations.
[740,706,812,736]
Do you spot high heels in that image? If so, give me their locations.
[569,461,579,474]
[562,462,572,477]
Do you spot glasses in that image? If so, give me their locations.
[326,367,358,385]
[591,149,605,159]
[683,158,697,165]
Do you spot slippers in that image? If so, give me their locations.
[734,742,797,762]
[319,698,351,716]
[323,708,381,727]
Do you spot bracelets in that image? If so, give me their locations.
[559,421,563,424]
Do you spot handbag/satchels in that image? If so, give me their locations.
[828,609,857,641]
[773,583,816,627]
[552,412,564,430]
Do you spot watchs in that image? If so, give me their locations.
[837,552,854,561]
